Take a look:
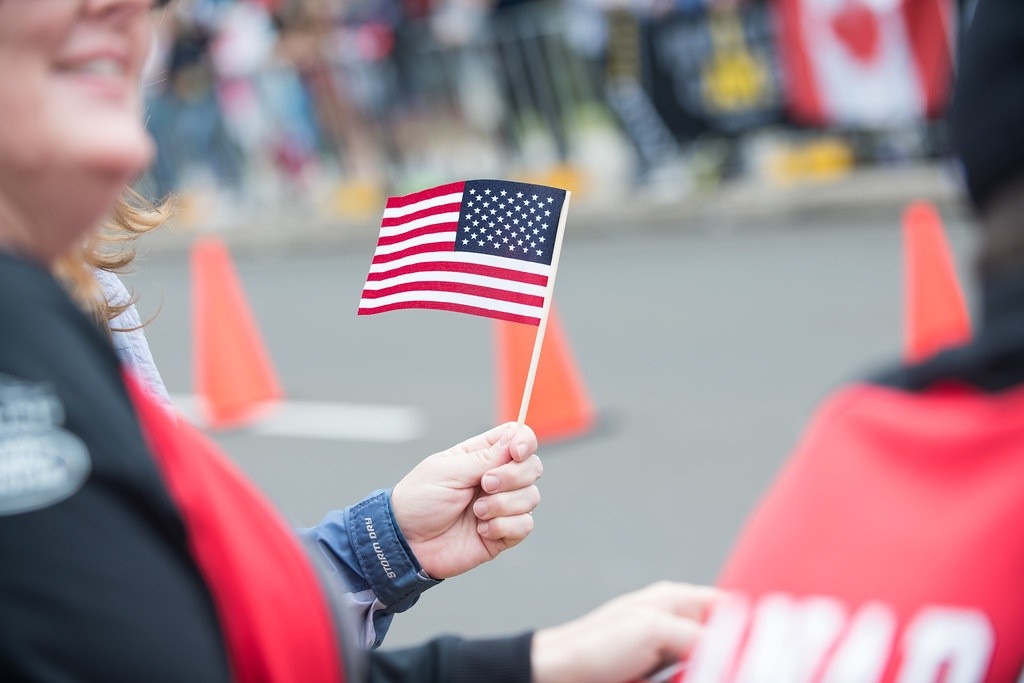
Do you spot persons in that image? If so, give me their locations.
[0,0,719,683]
[661,3,1024,681]
[118,0,980,218]
[56,190,542,642]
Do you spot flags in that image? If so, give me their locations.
[769,0,958,127]
[359,178,566,324]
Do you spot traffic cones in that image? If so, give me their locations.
[496,302,599,444]
[189,238,281,431]
[903,201,973,366]
